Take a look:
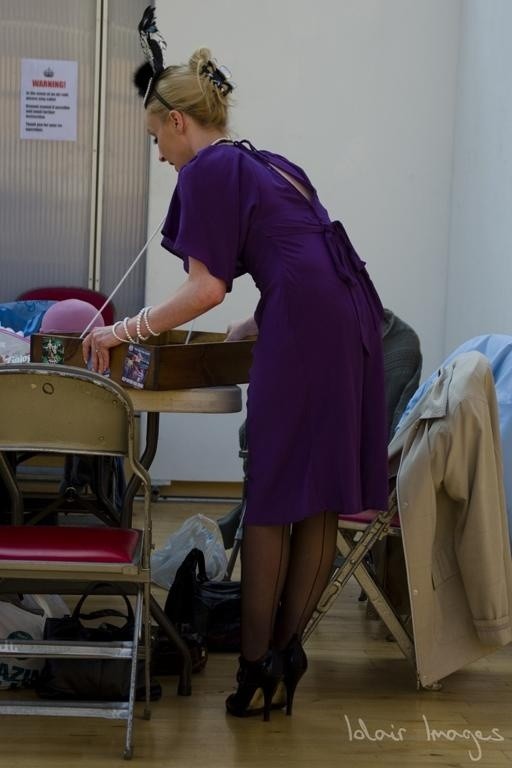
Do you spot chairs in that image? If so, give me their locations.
[0,353,165,763]
[279,346,498,691]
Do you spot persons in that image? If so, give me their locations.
[81,5,389,722]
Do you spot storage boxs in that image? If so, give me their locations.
[28,323,264,393]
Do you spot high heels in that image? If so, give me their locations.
[226,634,307,722]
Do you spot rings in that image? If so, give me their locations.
[95,349,102,352]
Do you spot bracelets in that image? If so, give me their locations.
[111,306,160,345]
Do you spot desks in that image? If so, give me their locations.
[1,375,251,703]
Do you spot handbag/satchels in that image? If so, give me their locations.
[122,622,208,674]
[37,617,161,703]
[163,570,285,654]
